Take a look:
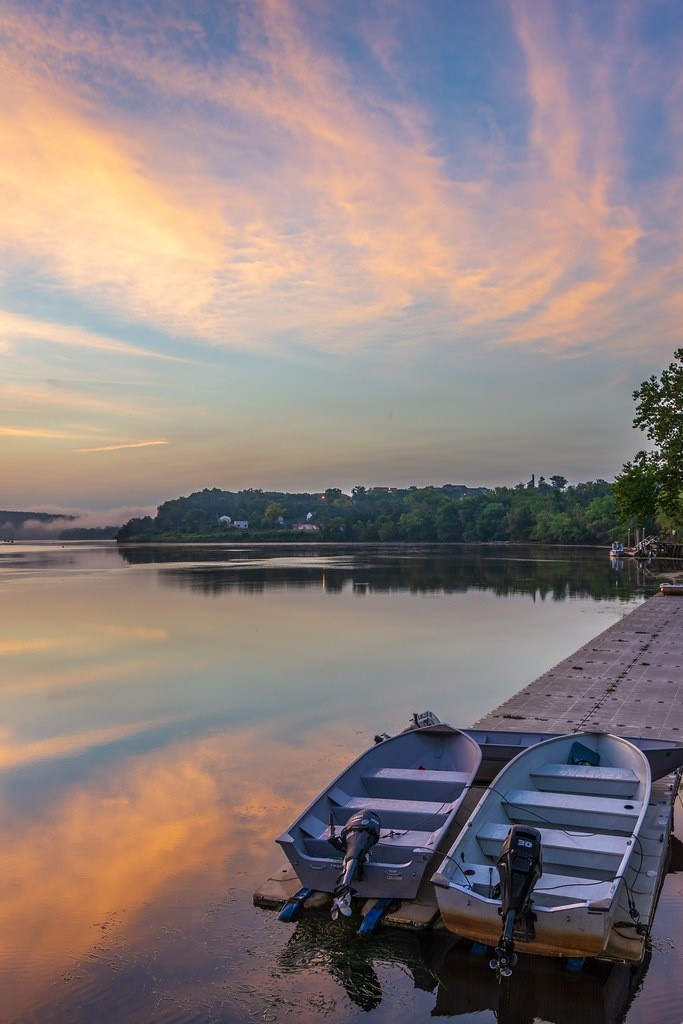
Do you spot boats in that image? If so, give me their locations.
[609,542,624,557]
[275,723,482,939]
[659,583,683,595]
[428,727,654,983]
